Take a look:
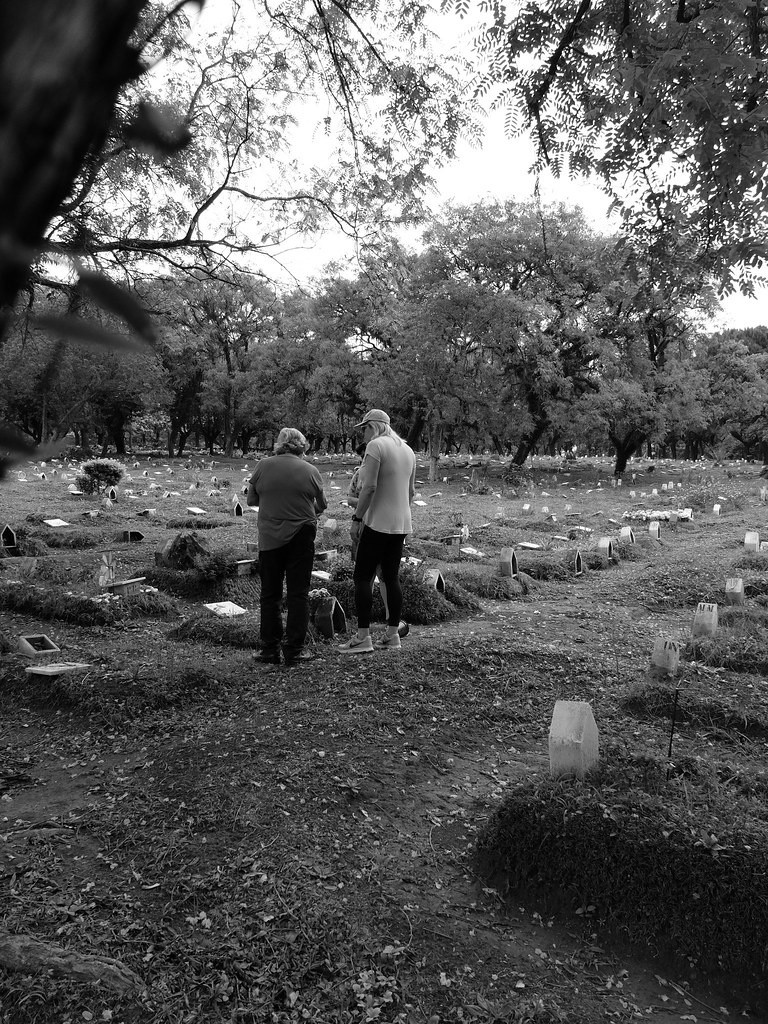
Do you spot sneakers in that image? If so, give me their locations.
[337,633,374,654]
[375,633,402,648]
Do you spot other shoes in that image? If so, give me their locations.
[252,649,280,665]
[285,646,313,666]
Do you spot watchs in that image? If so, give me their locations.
[352,515,363,523]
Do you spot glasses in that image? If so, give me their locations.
[361,425,368,432]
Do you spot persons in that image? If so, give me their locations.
[347,441,389,630]
[338,409,416,654]
[247,428,327,663]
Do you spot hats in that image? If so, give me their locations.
[354,409,390,431]
